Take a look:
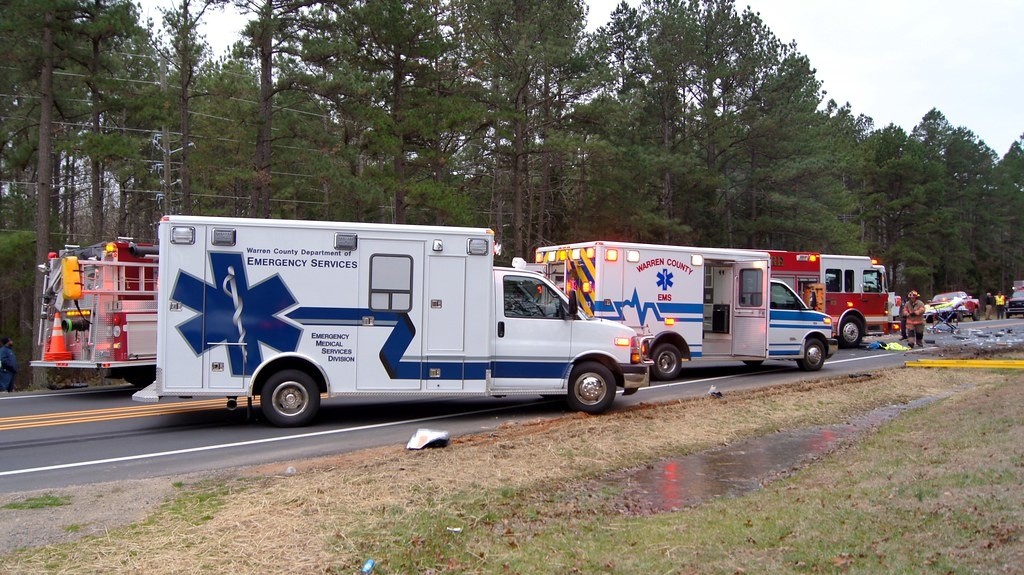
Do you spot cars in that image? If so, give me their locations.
[925,291,981,323]
[1005,285,1024,320]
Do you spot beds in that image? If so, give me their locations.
[922,304,969,332]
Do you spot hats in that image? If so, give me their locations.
[3,337,11,344]
[907,290,920,298]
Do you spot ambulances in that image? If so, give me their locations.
[525,239,839,382]
[128,216,657,417]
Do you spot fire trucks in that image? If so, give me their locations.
[29,235,159,388]
[745,249,902,349]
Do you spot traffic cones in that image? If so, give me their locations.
[43,312,73,362]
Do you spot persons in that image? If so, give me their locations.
[0,337,20,392]
[985,292,993,320]
[899,296,911,340]
[994,291,1005,319]
[903,290,926,349]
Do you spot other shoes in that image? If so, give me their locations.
[899,336,904,340]
[917,343,923,347]
[907,342,914,348]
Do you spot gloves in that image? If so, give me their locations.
[910,312,916,316]
